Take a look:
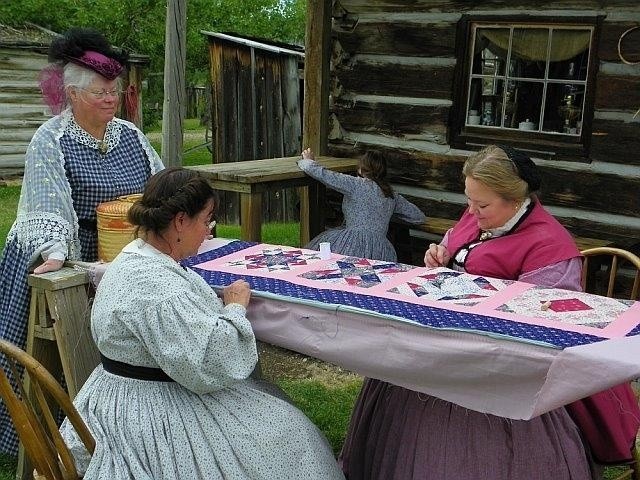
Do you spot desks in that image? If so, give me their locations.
[106,235,640,385]
[184,153,365,251]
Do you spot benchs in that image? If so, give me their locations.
[393,211,611,281]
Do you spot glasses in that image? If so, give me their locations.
[75,84,122,98]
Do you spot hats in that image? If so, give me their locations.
[37,29,131,116]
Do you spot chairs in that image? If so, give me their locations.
[577,246,640,470]
[0,340,99,479]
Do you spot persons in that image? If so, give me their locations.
[340,143,640,480]
[297,147,427,264]
[35,167,348,479]
[0,26,169,455]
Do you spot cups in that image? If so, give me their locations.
[467,116,480,127]
[519,118,537,130]
[318,242,331,260]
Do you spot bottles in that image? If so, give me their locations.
[562,119,572,133]
